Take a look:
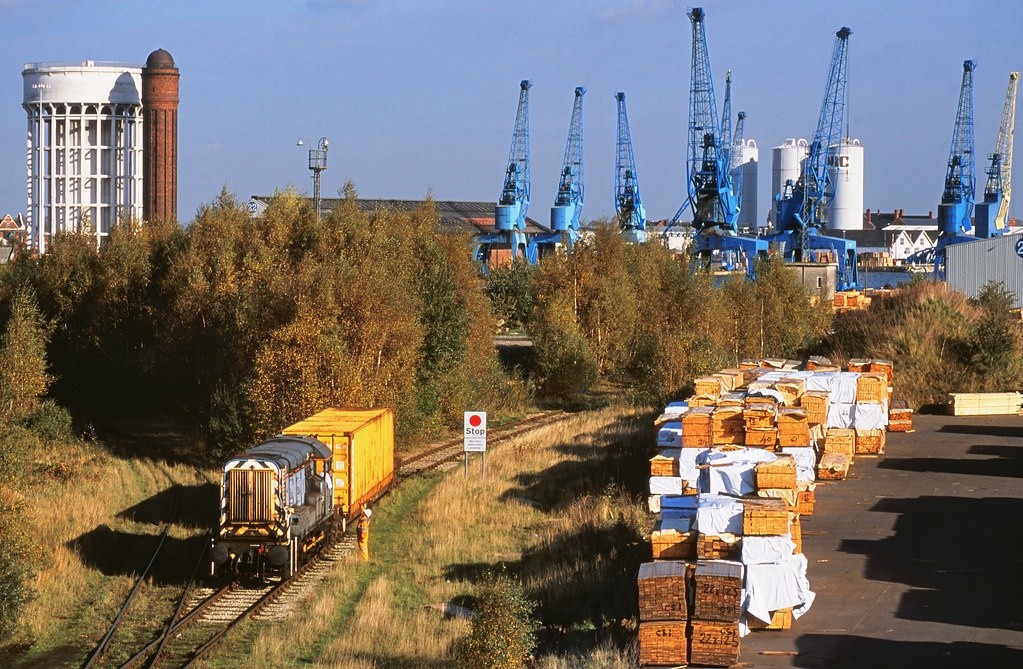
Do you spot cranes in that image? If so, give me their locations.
[663,5,772,285]
[760,27,860,291]
[467,79,535,276]
[614,91,652,243]
[896,59,1021,282]
[526,84,588,267]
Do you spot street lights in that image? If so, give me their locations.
[297,136,330,220]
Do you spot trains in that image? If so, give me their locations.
[208,405,397,588]
